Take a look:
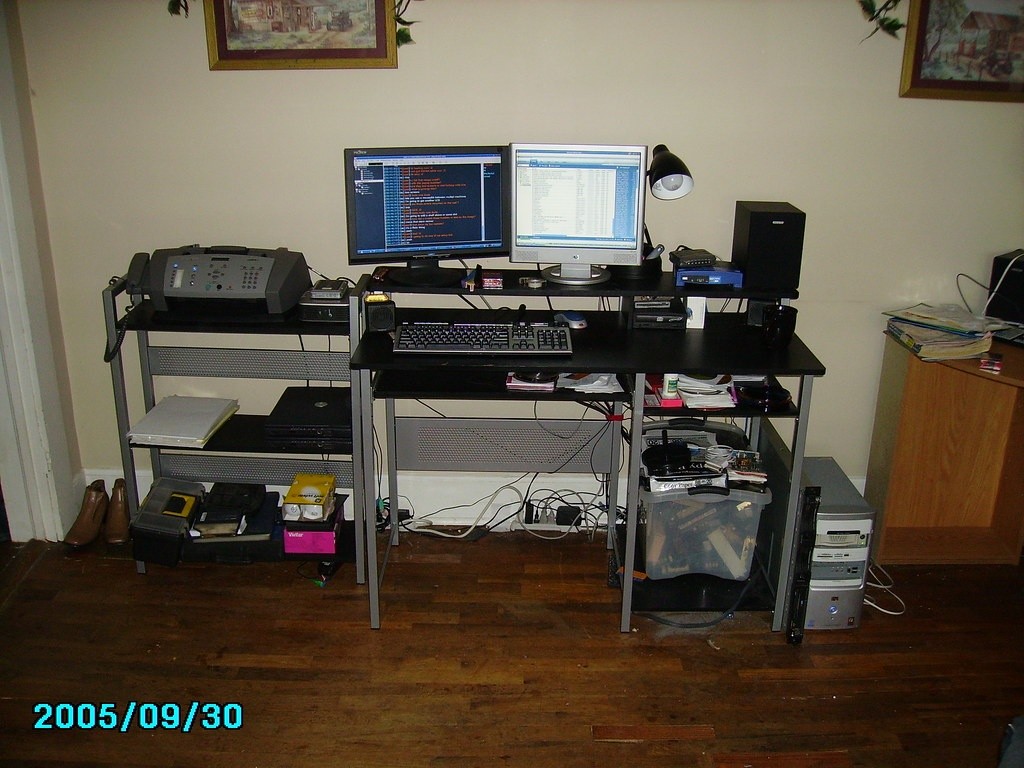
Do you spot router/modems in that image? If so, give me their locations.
[669,249,717,268]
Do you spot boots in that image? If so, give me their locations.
[105,478,131,546]
[63,479,109,549]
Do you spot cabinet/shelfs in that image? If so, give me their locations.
[102,274,372,584]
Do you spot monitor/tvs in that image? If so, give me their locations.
[342,143,646,285]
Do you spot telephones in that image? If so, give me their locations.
[126,252,150,295]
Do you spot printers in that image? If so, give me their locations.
[135,244,312,322]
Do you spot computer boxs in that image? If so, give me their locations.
[788,457,878,631]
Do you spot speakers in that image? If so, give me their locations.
[364,300,396,332]
[747,300,776,327]
[731,200,806,296]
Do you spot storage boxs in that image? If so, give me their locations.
[128,476,204,569]
[282,473,344,554]
[980,352,1002,370]
[481,270,503,287]
[639,485,773,581]
[655,387,682,407]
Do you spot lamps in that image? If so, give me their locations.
[608,144,694,279]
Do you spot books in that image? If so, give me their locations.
[505,372,554,391]
[645,374,766,408]
[191,490,279,542]
[881,302,1011,361]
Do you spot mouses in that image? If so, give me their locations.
[554,310,587,329]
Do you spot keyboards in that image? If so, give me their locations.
[393,323,573,356]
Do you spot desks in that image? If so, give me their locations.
[864,330,1024,567]
[349,266,826,633]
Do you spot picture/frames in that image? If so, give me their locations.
[202,0,398,71]
[898,0,1024,105]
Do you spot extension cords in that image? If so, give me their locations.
[511,517,582,534]
[376,520,417,531]
